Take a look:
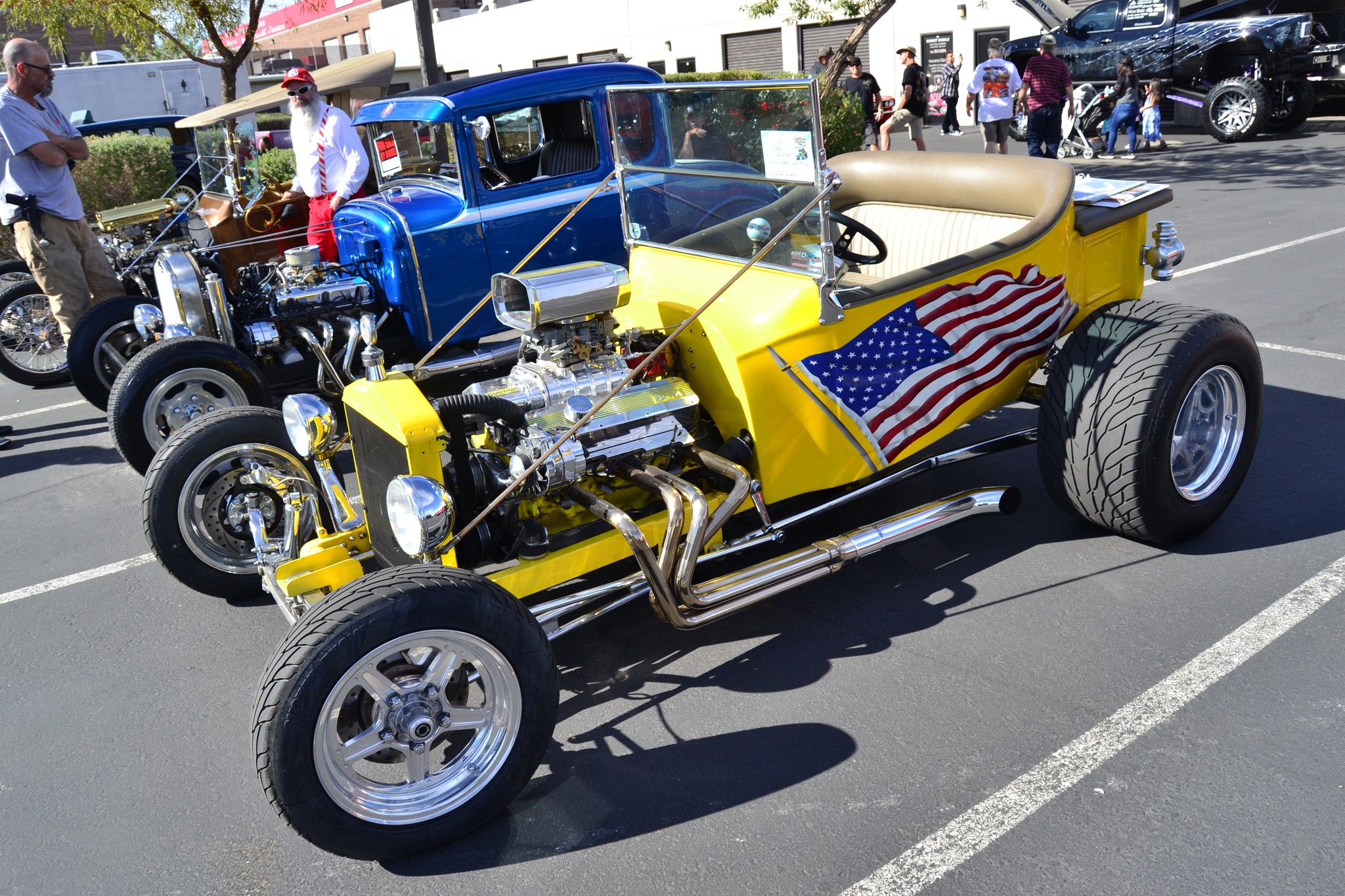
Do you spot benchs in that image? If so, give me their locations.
[1072,173,1175,240]
[538,140,601,178]
[828,200,1036,287]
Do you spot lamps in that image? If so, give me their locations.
[957,5,966,18]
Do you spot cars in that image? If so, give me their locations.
[43,106,229,219]
[68,62,787,478]
[0,48,403,388]
[868,94,895,129]
[138,73,1264,859]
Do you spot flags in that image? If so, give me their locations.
[795,262,1084,476]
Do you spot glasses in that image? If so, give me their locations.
[287,85,313,99]
[14,62,55,76]
[850,64,862,67]
[945,50,952,57]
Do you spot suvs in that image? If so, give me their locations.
[990,0,1315,142]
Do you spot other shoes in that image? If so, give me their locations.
[940,130,949,136]
[1120,153,1136,158]
[1155,141,1167,151]
[1097,152,1116,159]
[1139,144,1151,151]
[949,130,964,135]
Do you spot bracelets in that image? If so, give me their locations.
[879,109,884,112]
[966,106,971,109]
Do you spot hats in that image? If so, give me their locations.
[896,46,917,58]
[818,45,835,59]
[1039,34,1056,45]
[281,67,314,88]
[850,57,862,63]
[987,37,1003,49]
[1120,55,1133,66]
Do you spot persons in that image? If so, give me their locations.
[1079,101,1090,117]
[842,57,885,152]
[680,104,747,167]
[1098,54,1135,160]
[809,45,838,92]
[257,137,272,156]
[235,130,255,194]
[935,78,943,88]
[1139,78,1169,152]
[1125,87,1145,151]
[1017,33,1075,160]
[1,37,128,356]
[965,38,1029,155]
[878,44,929,152]
[939,50,964,136]
[279,69,368,270]
